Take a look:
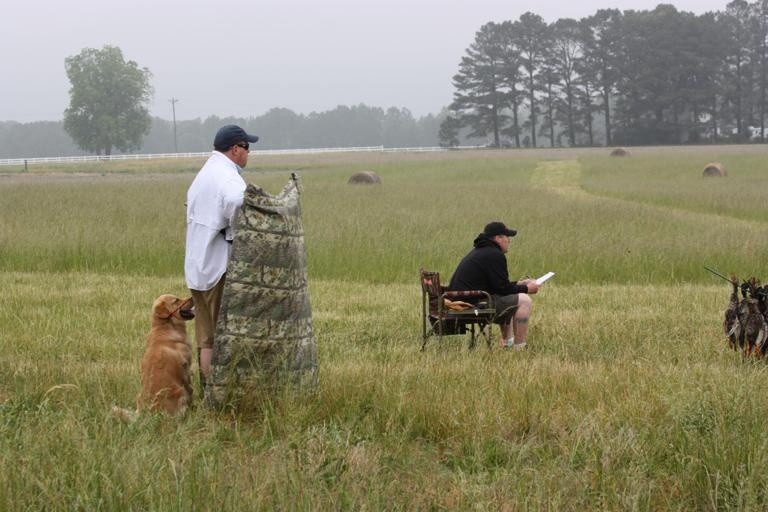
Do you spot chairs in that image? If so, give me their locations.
[420,269,498,355]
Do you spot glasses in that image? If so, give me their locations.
[238,144,249,150]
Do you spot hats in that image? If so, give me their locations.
[484,222,516,236]
[214,125,258,148]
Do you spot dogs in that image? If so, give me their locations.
[112,294,196,426]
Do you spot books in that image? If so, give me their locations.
[535,269,556,285]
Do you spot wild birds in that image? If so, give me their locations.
[723,273,768,364]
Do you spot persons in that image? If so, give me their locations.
[449,222,538,350]
[185,124,259,388]
